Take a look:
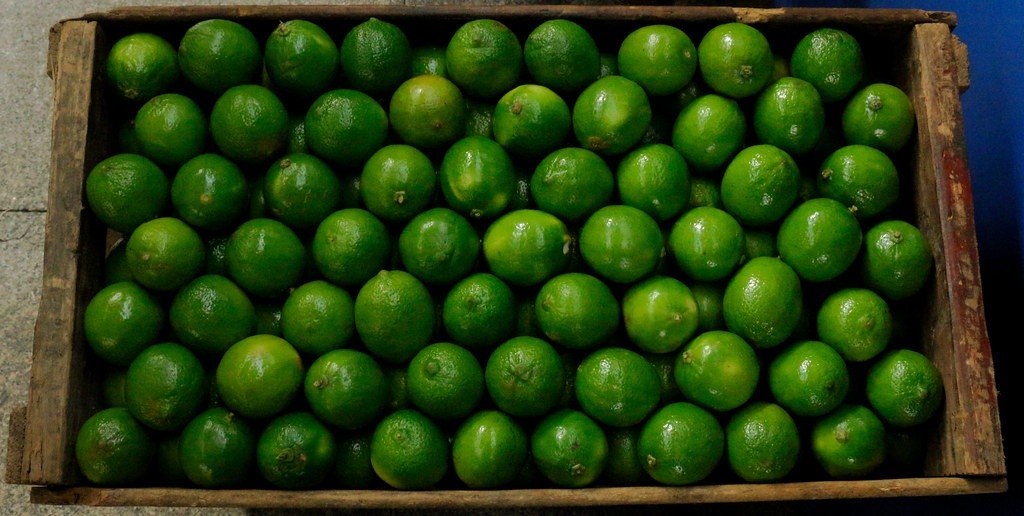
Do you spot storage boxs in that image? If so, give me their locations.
[4,1,1012,515]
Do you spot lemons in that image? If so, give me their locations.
[75,16,946,489]
